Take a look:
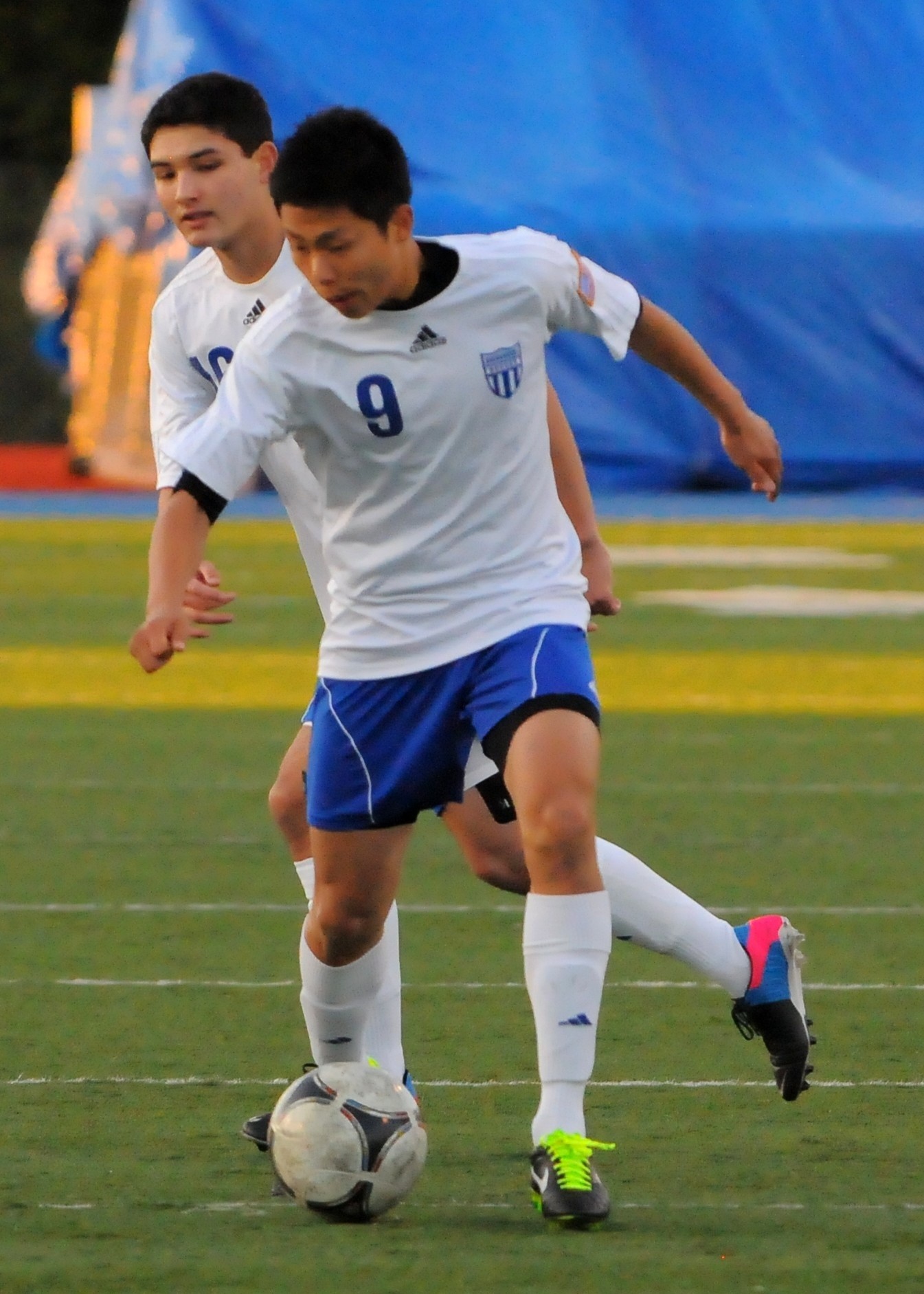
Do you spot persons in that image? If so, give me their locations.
[129,105,780,1230]
[141,71,820,1101]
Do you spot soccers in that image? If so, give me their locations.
[267,1062,428,1222]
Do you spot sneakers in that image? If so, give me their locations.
[529,1131,616,1222]
[732,915,817,1102]
[403,1069,419,1105]
[241,1064,319,1152]
